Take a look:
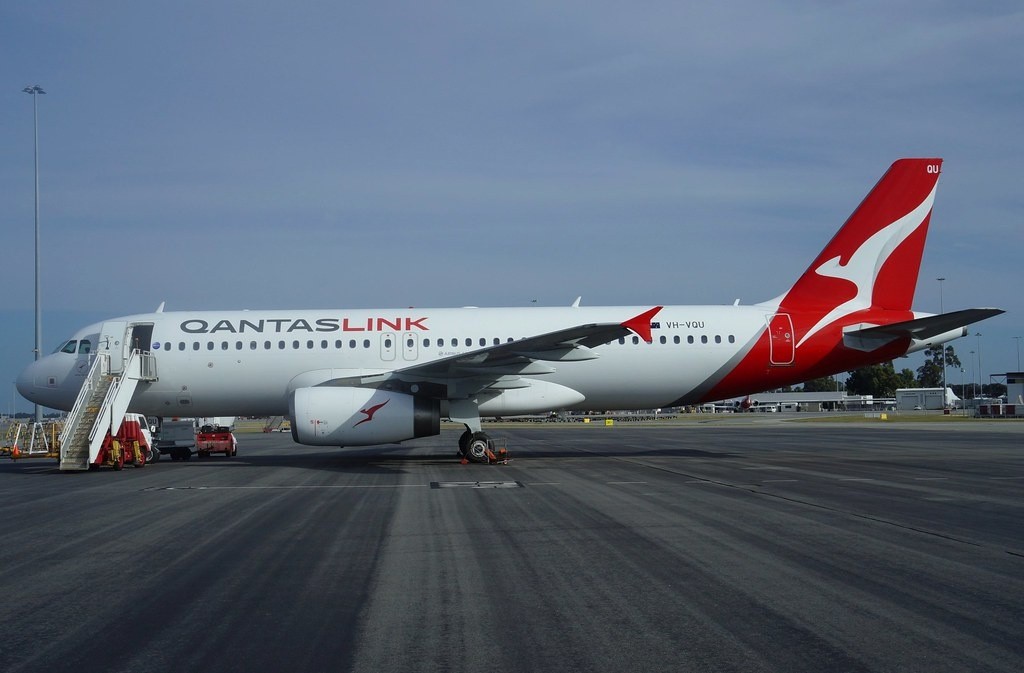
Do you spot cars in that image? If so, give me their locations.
[197,424,237,458]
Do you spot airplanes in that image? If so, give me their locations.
[17,157,1010,463]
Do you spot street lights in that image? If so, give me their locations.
[23,84,47,425]
